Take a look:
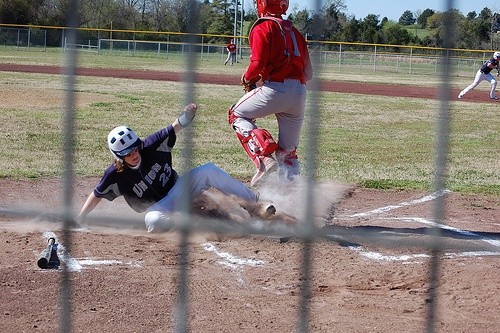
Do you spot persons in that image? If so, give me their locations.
[224,39,236,66]
[458,52,500,100]
[73,102,276,234]
[229,0,313,190]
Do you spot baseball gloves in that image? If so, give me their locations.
[240,73,262,93]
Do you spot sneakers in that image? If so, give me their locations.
[248,192,276,219]
[277,171,299,196]
[251,154,279,188]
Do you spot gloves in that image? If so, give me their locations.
[178,102,197,128]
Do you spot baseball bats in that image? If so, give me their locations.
[37,238,56,268]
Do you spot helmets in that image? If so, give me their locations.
[493,52,500,60]
[108,125,142,163]
[257,0,289,16]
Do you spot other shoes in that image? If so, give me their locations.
[458,94,462,99]
[490,96,499,100]
[224,62,226,65]
[231,64,233,66]
